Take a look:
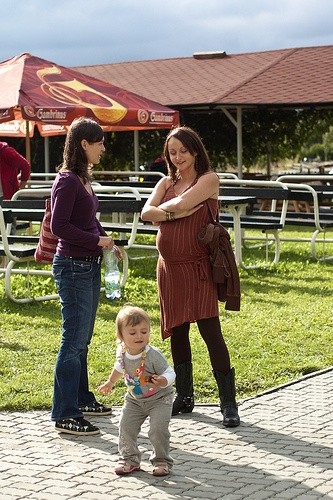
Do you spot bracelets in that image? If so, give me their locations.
[165,211,175,222]
[110,379,115,384]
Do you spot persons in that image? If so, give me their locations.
[97,306,177,477]
[34,116,122,436]
[140,127,241,427]
[0,141,31,268]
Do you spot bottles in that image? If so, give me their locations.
[102,245,122,299]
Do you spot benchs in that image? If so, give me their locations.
[0,244,36,257]
[0,235,128,247]
[220,214,333,228]
[248,210,333,220]
[215,220,284,231]
[101,221,158,235]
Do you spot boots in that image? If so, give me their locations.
[171,363,195,415]
[211,367,241,427]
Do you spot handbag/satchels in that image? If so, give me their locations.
[33,198,59,263]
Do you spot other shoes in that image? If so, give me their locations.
[152,462,170,476]
[114,462,140,475]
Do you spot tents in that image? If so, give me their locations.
[0,53,183,188]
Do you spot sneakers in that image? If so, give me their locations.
[54,417,100,435]
[78,393,113,415]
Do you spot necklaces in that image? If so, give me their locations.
[120,344,147,385]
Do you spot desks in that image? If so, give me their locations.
[0,172,333,300]
